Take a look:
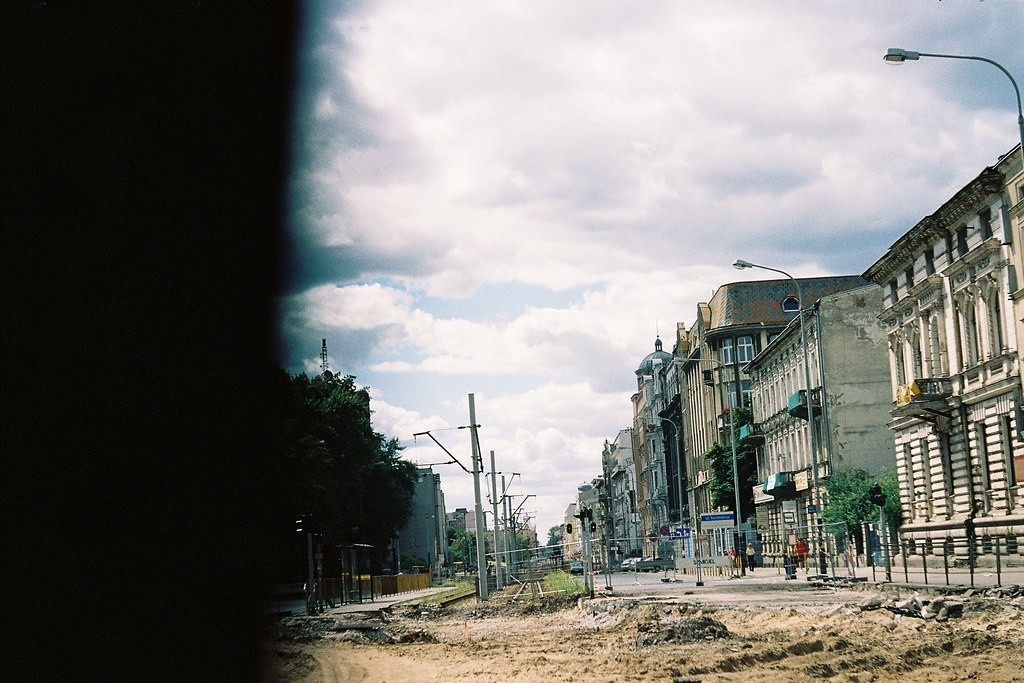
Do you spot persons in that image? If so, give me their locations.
[728,547,736,565]
[796,538,809,572]
[746,543,755,572]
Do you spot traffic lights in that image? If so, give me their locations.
[591,522,596,532]
[566,523,572,533]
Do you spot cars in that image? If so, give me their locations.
[621,557,674,571]
[571,562,583,573]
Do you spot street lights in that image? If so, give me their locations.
[733,259,826,574]
[605,465,638,554]
[618,446,656,572]
[637,416,686,574]
[883,48,1023,197]
[674,357,746,576]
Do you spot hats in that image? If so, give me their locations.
[748,543,752,545]
